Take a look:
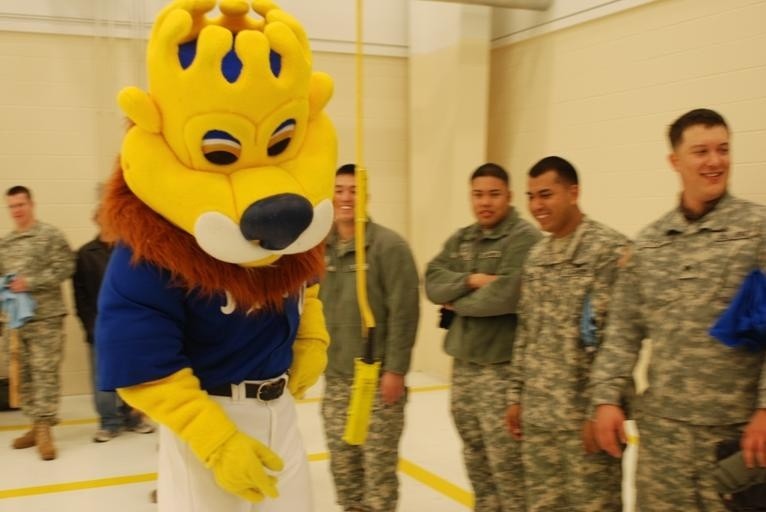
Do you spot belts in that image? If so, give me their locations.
[203,370,291,400]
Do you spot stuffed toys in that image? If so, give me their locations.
[92,1,337,512]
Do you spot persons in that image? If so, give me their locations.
[74,232,155,443]
[0,307,23,412]
[315,165,420,512]
[0,186,77,460]
[582,110,766,512]
[425,165,545,512]
[504,157,644,512]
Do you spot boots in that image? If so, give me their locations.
[14,427,35,448]
[94,430,119,442]
[34,421,55,460]
[121,422,154,433]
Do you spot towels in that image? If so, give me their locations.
[0,272,39,329]
